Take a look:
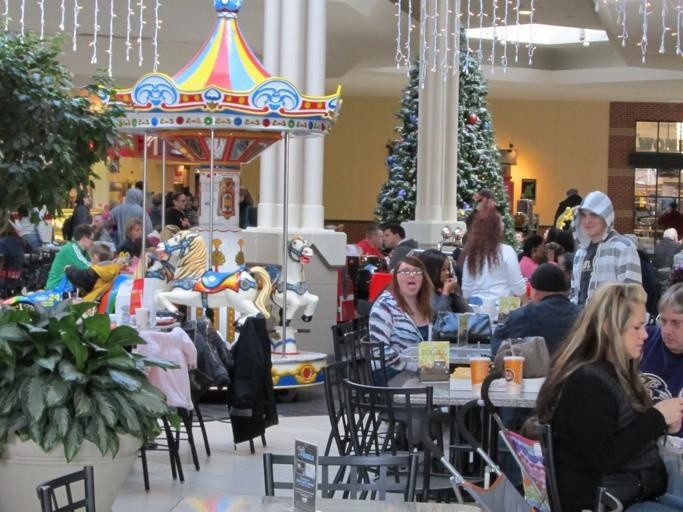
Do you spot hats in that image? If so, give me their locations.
[528,262,568,293]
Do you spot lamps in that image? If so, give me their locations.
[463,23,609,45]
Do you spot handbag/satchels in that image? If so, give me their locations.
[431,310,492,344]
[494,336,551,379]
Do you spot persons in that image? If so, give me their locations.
[490,263,580,362]
[658,202,683,241]
[553,188,583,228]
[381,223,418,273]
[518,235,555,281]
[238,185,254,229]
[536,282,683,511]
[116,217,143,257]
[63,244,120,301]
[72,189,93,229]
[623,234,660,315]
[457,208,528,311]
[544,227,575,270]
[653,228,682,268]
[419,249,475,325]
[354,224,384,261]
[634,282,683,440]
[568,191,643,311]
[462,190,495,248]
[165,190,191,230]
[46,225,100,290]
[135,180,150,197]
[92,215,112,243]
[104,188,154,241]
[369,256,442,393]
[184,191,199,225]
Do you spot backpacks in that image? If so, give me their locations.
[61,216,73,242]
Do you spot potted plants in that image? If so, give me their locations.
[0,303,183,512]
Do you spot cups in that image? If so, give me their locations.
[503,355,524,398]
[471,358,489,400]
[483,298,496,324]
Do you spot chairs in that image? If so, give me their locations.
[35,466,95,512]
[131,327,183,491]
[360,334,425,424]
[341,326,368,455]
[39,251,56,290]
[322,320,365,463]
[179,316,212,470]
[527,424,561,512]
[174,314,266,454]
[343,379,484,503]
[263,452,418,502]
[320,361,434,500]
[24,251,40,291]
[0,255,26,299]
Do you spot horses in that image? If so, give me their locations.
[257,237,321,327]
[156,229,273,330]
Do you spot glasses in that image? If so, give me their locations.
[395,269,423,275]
[654,313,683,330]
